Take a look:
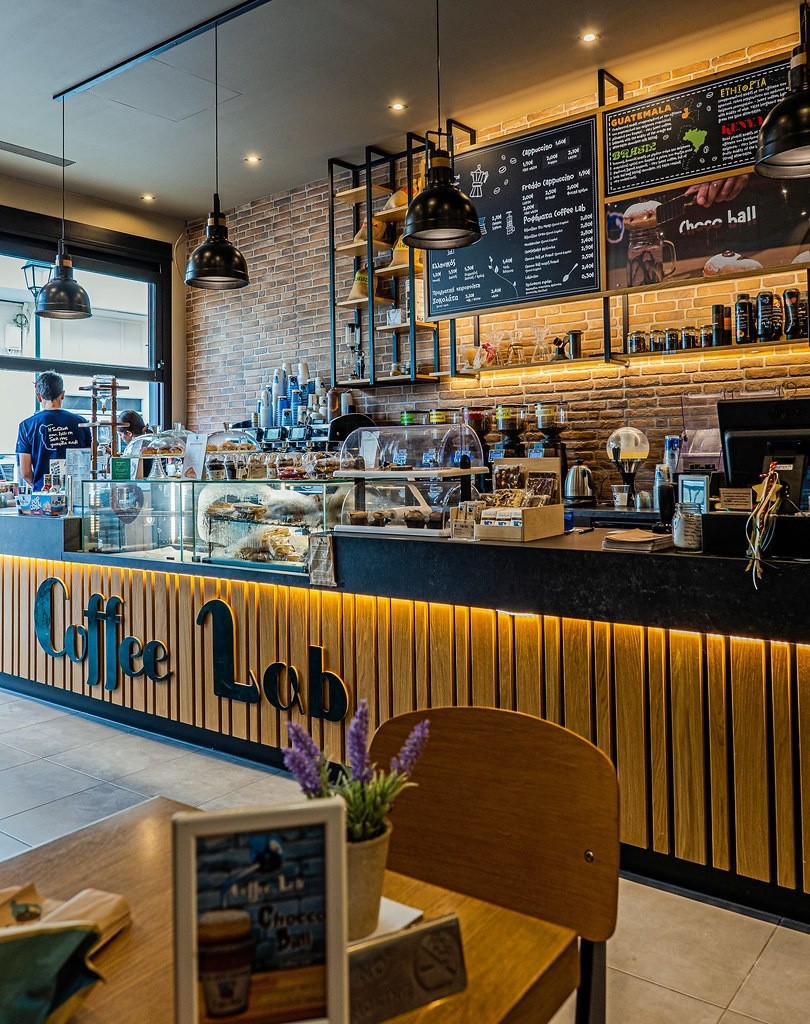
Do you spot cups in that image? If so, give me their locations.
[203,451,355,483]
[627,230,677,288]
[252,362,329,430]
[611,484,630,508]
[651,435,684,512]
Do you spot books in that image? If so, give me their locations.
[602,528,674,554]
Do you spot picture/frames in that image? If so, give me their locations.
[173,795,349,1021]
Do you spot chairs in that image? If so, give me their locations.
[368,706,619,1024]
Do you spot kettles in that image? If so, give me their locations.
[563,458,597,506]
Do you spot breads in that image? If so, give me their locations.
[209,501,300,563]
[623,200,663,228]
[791,248,810,263]
[140,442,255,454]
[703,250,762,277]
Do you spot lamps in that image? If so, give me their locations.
[402,0,480,250]
[33,94,95,320]
[185,22,250,291]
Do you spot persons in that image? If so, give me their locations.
[685,174,750,208]
[15,371,92,492]
[99,411,153,477]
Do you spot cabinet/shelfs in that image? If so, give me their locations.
[330,186,438,390]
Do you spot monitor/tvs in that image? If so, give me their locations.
[718,399,810,514]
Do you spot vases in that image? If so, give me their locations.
[344,813,393,941]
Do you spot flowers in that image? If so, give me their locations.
[286,700,430,840]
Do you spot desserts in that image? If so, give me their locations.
[348,510,448,530]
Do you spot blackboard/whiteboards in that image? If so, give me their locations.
[424,106,601,324]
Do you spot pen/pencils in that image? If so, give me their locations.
[579,527,594,534]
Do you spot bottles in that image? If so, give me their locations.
[567,329,582,360]
[0,480,32,508]
[506,342,527,366]
[326,388,340,423]
[624,287,809,355]
[671,502,703,553]
[40,473,66,494]
[340,392,353,416]
[530,340,551,364]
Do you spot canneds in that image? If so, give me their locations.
[626,325,713,354]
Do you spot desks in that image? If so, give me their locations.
[0,796,583,1024]
